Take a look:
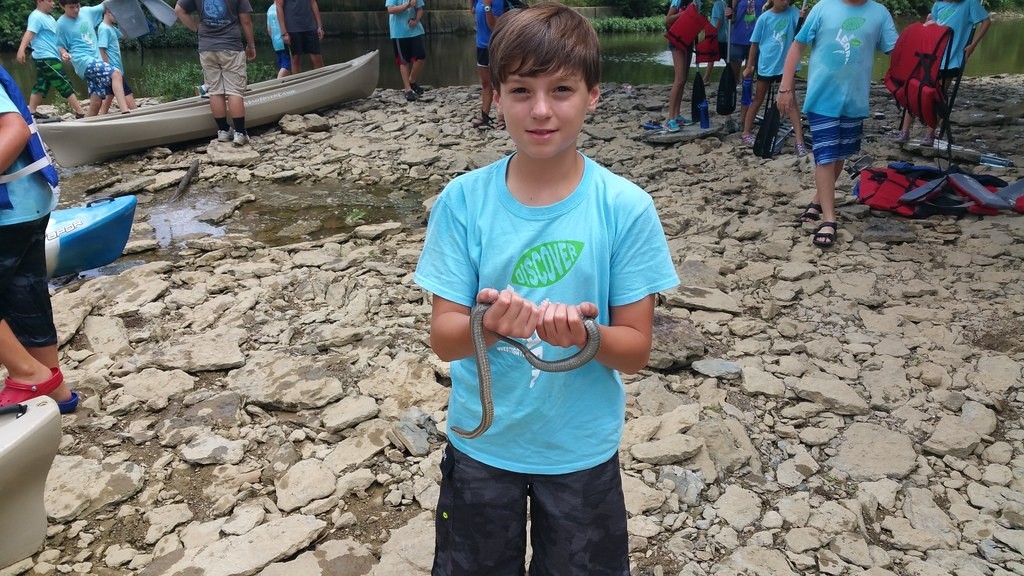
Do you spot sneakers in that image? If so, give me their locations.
[676,115,694,126]
[218,126,234,142]
[919,133,934,146]
[665,118,680,133]
[233,130,250,145]
[794,142,809,156]
[741,134,757,148]
[410,81,424,95]
[405,90,416,101]
[893,131,910,143]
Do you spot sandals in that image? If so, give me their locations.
[798,201,822,222]
[496,119,506,130]
[470,116,487,128]
[0,366,80,414]
[813,221,838,247]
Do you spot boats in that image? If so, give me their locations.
[35,49,381,169]
[45,194,138,281]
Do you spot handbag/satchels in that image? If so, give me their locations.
[753,104,780,158]
[717,64,737,115]
[691,72,706,122]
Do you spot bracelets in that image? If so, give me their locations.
[317,25,324,31]
[778,89,797,94]
[282,32,288,36]
[482,3,490,13]
[245,44,255,49]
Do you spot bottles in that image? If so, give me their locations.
[741,77,752,105]
[699,99,709,129]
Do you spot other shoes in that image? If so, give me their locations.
[30,112,50,120]
[194,85,206,96]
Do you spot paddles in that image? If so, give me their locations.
[753,1,810,158]
[691,0,707,122]
[716,1,738,115]
[938,0,985,140]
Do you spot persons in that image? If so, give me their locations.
[0,65,80,414]
[892,0,991,147]
[414,0,684,576]
[663,0,810,158]
[777,0,937,247]
[384,0,428,102]
[18,0,139,119]
[470,0,529,133]
[173,0,257,147]
[266,1,326,79]
